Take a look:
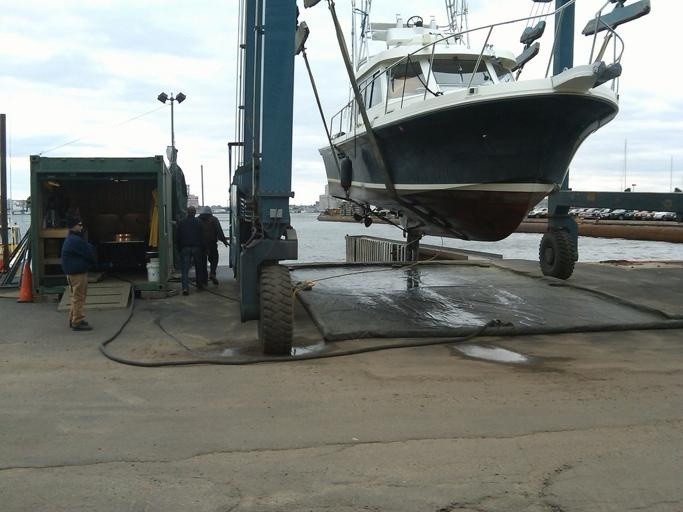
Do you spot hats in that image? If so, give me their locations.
[199,205,212,215]
[67,214,81,228]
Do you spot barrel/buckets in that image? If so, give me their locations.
[146,264,160,282]
[149,257,159,263]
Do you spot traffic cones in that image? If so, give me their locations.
[16,262,34,303]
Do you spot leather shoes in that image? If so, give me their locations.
[69,319,92,331]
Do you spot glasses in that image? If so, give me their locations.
[77,224,83,226]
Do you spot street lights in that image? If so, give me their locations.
[159,91,186,219]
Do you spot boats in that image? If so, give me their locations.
[319,0,627,241]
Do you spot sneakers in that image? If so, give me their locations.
[182,286,190,296]
[195,276,208,289]
[209,275,218,286]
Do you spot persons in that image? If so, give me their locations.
[61,217,90,332]
[196,206,229,284]
[174,204,206,295]
